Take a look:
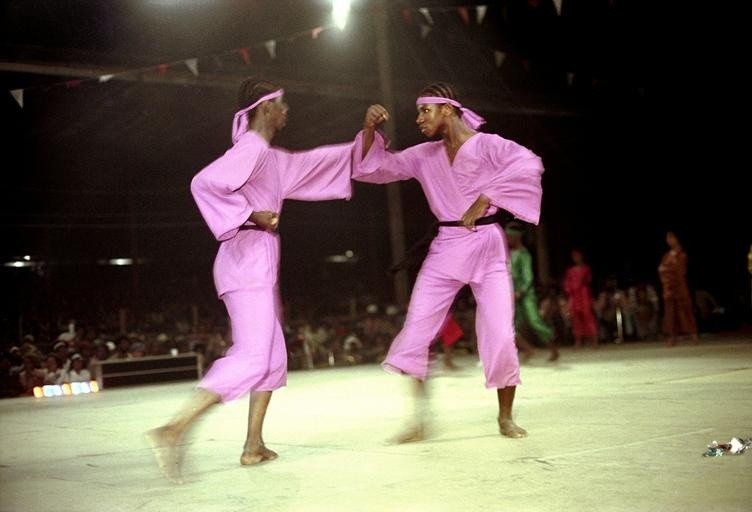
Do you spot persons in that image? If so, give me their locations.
[351,82,545,446]
[281,302,405,370]
[506,225,697,368]
[148,77,391,486]
[429,297,479,370]
[0,319,234,396]
